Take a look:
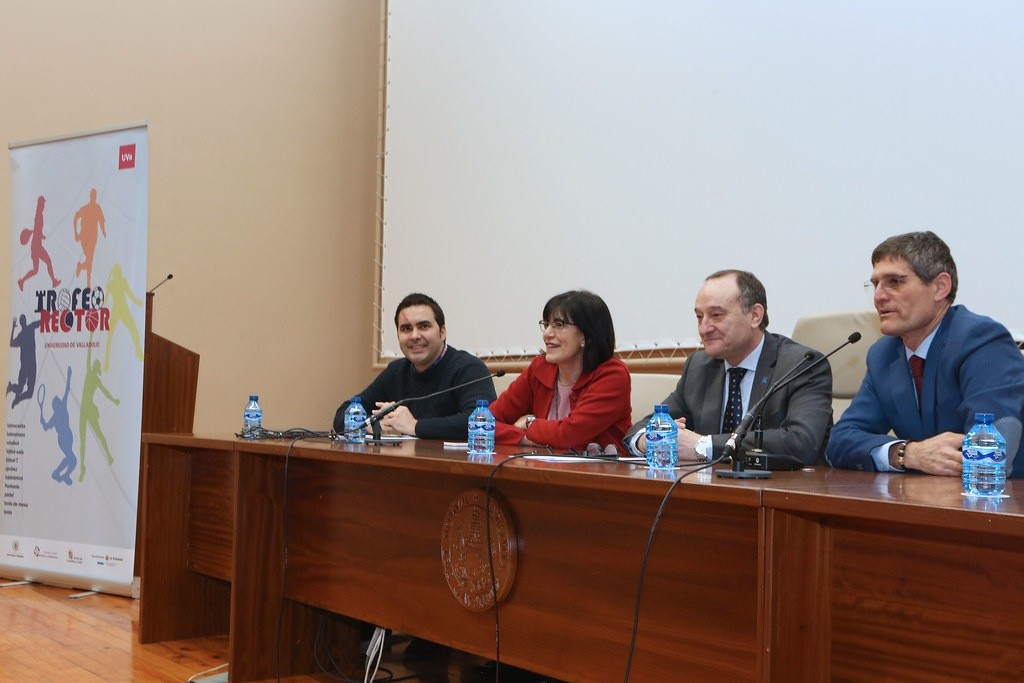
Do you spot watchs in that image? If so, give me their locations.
[694,435,708,461]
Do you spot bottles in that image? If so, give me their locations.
[961,413,1007,496]
[344,397,367,443]
[244,395,262,438]
[468,400,495,454]
[646,404,678,469]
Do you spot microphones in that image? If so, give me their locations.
[150,274,173,293]
[714,332,862,478]
[353,370,507,444]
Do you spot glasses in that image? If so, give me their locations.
[539,319,577,333]
[864,274,917,294]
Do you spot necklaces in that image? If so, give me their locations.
[554,382,559,420]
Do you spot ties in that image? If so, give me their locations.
[909,354,926,418]
[721,367,748,434]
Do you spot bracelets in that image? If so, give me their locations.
[899,439,911,470]
[525,415,536,428]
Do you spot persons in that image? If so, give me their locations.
[622,269,835,471]
[484,291,633,458]
[825,229,1024,480]
[333,294,498,440]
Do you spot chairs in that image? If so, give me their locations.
[790,311,897,438]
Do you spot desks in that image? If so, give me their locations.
[229,435,962,683]
[762,477,1024,683]
[137,431,333,683]
